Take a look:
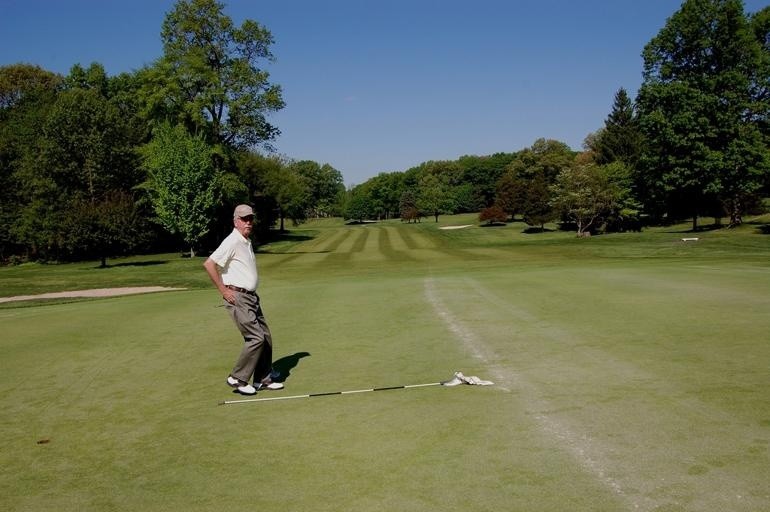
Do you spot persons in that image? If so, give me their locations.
[202,204,285,396]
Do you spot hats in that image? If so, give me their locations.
[233,205,256,218]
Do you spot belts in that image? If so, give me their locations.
[226,285,255,294]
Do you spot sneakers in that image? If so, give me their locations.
[227,375,256,395]
[253,378,284,390]
